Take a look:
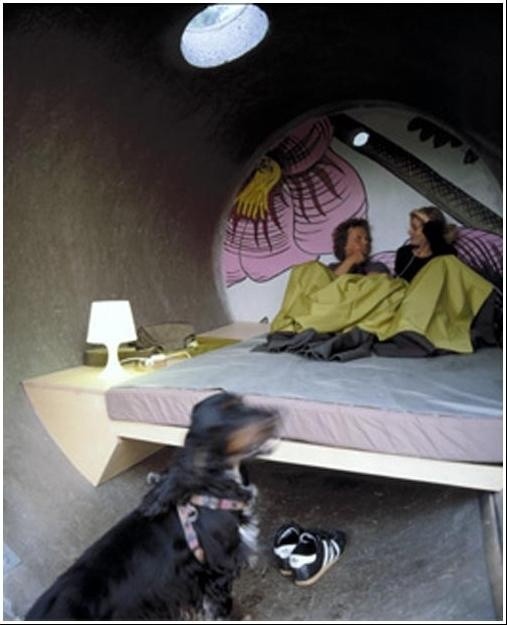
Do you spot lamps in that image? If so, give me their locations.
[85,300,139,384]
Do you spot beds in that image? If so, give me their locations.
[104,329,504,494]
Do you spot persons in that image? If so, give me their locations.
[390,203,467,282]
[326,215,392,279]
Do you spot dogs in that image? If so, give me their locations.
[23,391,281,623]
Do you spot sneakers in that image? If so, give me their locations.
[289,530,347,587]
[272,522,306,577]
